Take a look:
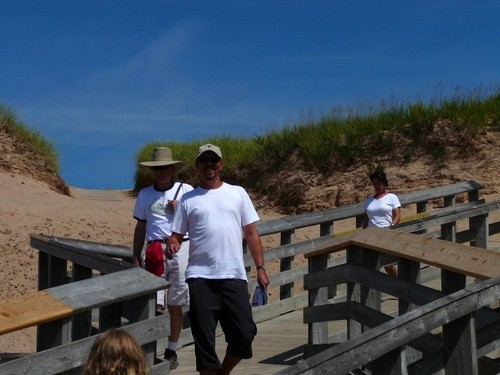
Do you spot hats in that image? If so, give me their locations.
[197,144,223,160]
[139,147,182,166]
[145,242,164,276]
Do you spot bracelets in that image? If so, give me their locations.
[256,266,267,271]
[391,223,395,226]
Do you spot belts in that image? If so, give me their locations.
[148,238,189,244]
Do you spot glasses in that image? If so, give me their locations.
[198,157,221,164]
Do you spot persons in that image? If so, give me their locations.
[132,147,194,369]
[361,171,402,278]
[85,329,153,375]
[165,144,270,375]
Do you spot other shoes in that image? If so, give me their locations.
[163,348,179,370]
